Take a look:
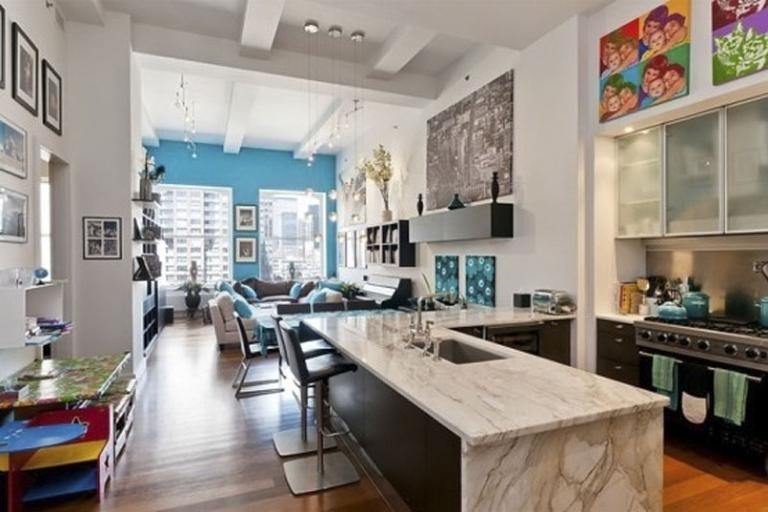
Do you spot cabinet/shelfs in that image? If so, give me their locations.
[365,220,416,268]
[596,314,652,389]
[132,200,174,357]
[0,281,63,348]
[615,93,768,240]
[0,352,138,512]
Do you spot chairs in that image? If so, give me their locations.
[209,291,278,353]
[230,300,378,499]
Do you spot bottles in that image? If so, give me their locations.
[678,275,690,293]
[448,193,464,211]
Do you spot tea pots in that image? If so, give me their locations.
[657,287,687,320]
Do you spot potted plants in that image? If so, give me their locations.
[174,281,210,308]
[361,144,393,222]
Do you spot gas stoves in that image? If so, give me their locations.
[644,316,768,339]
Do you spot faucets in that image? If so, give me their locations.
[405,292,452,355]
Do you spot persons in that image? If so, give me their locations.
[599,4,687,124]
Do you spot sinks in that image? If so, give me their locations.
[410,337,512,366]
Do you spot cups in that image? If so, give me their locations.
[646,298,658,315]
[625,224,638,235]
[640,218,650,233]
[639,304,650,316]
[754,296,768,327]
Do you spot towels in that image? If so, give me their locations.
[654,354,747,427]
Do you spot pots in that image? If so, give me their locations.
[682,292,710,318]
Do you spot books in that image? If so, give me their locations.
[25,315,74,346]
[366,228,399,266]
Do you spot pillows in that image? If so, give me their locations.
[214,281,257,321]
[290,280,345,303]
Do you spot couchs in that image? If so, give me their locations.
[234,277,314,302]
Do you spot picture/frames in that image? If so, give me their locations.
[83,217,122,260]
[0,5,62,244]
[235,204,258,264]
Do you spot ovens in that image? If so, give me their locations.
[638,347,768,477]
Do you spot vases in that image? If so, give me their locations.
[381,210,392,222]
